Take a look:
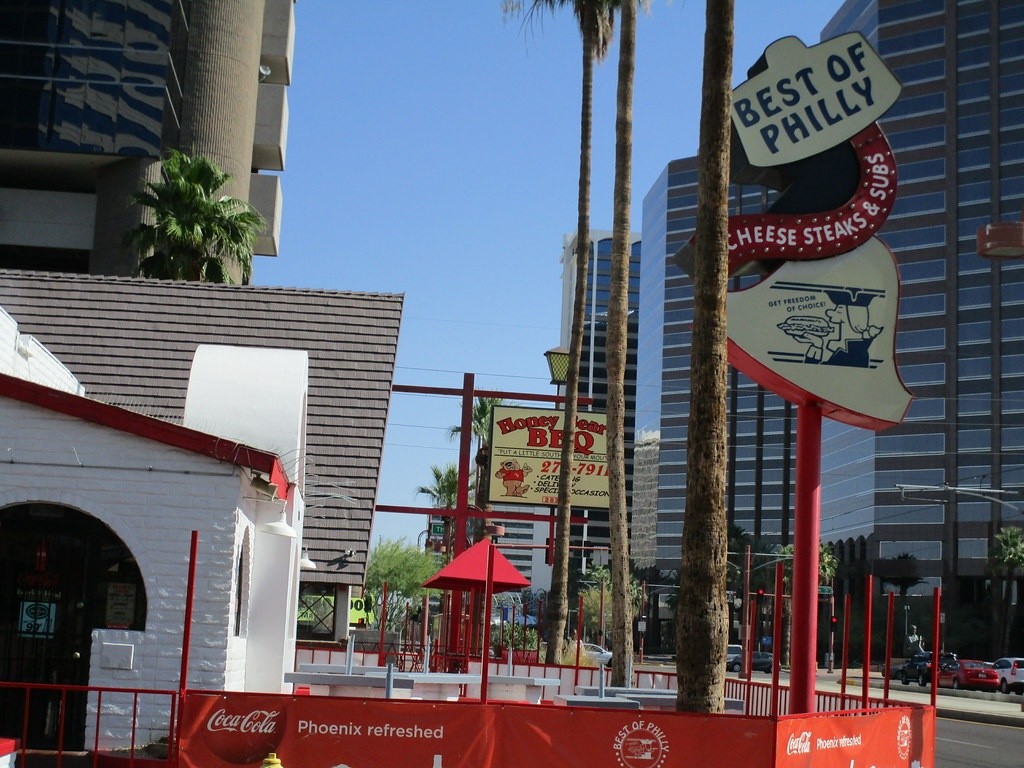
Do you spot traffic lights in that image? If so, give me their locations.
[757,582,766,599]
[358,618,364,628]
[830,616,838,632]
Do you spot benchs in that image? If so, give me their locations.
[283,672,416,698]
[554,695,640,708]
[615,693,744,714]
[575,686,678,698]
[365,671,482,701]
[464,673,561,704]
[298,663,399,675]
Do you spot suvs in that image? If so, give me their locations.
[901,651,960,686]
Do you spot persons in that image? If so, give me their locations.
[563,627,585,656]
[903,624,925,658]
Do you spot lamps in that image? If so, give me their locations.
[300,546,317,570]
[243,496,298,538]
[345,547,357,558]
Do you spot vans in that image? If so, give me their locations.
[726,644,743,671]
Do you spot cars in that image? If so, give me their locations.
[729,651,781,674]
[938,659,1001,693]
[882,660,910,681]
[990,657,1024,695]
[582,642,613,668]
